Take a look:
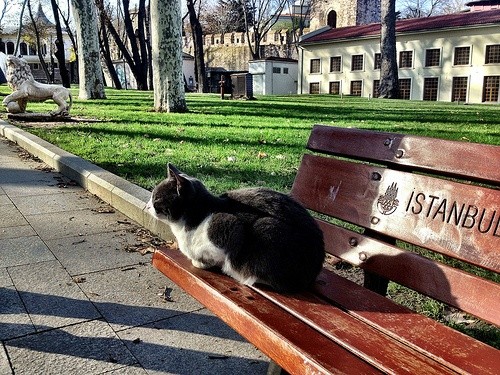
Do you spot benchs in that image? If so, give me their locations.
[151,125,500,375]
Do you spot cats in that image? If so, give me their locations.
[146,162,326,289]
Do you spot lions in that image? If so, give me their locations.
[2,54,73,115]
